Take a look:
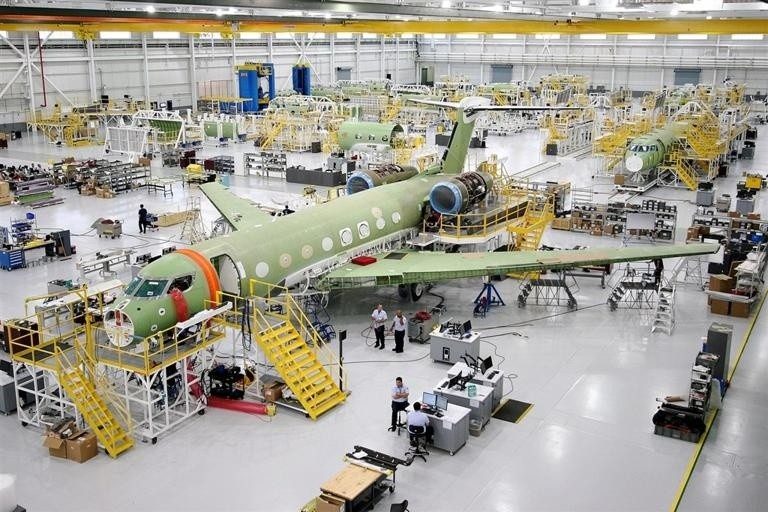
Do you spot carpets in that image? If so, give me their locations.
[493,398,534,424]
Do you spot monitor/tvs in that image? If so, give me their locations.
[448,376,458,389]
[422,392,436,408]
[477,355,493,369]
[457,371,462,382]
[439,317,471,339]
[434,395,448,412]
[471,361,487,375]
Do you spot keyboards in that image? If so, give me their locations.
[441,381,449,388]
[488,372,495,379]
[465,333,472,338]
[421,408,436,415]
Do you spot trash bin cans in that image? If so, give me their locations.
[469,419,482,436]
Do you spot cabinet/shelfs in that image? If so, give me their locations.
[161,148,287,187]
[686,214,768,253]
[570,199,677,243]
[52,158,152,195]
[690,361,713,411]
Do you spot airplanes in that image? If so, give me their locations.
[100,97,723,352]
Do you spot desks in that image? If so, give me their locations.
[445,363,504,409]
[146,178,174,197]
[429,326,480,365]
[405,397,471,456]
[434,378,494,432]
[320,463,386,512]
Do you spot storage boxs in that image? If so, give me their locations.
[302,496,345,512]
[708,273,753,318]
[551,218,572,229]
[264,383,281,401]
[66,428,98,462]
[42,419,79,457]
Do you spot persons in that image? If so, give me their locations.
[0,159,97,194]
[390,309,407,353]
[371,305,388,349]
[652,259,664,286]
[391,376,410,431]
[138,204,147,233]
[406,402,434,444]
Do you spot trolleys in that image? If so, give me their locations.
[407,306,443,345]
[162,151,178,168]
[97,218,124,239]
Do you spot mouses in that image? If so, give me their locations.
[475,333,478,335]
[495,372,498,374]
[438,388,441,389]
[438,414,441,417]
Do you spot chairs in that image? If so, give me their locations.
[390,500,409,512]
[404,425,429,462]
[387,403,409,435]
